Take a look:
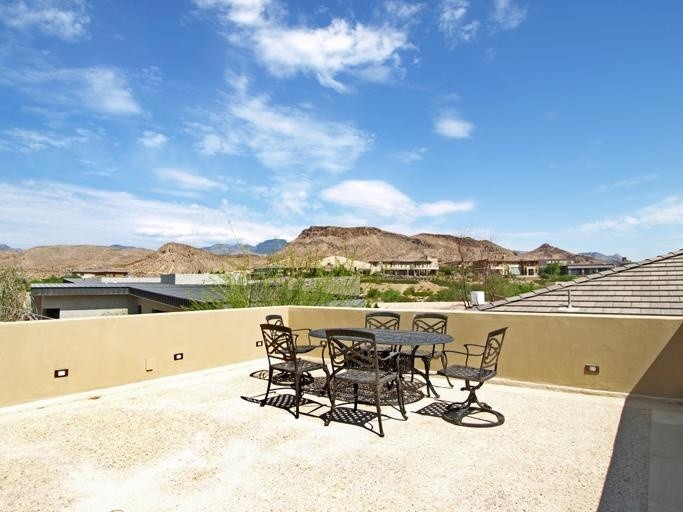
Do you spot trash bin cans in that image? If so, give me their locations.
[471,291,485,306]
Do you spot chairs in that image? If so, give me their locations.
[259,312,508,438]
